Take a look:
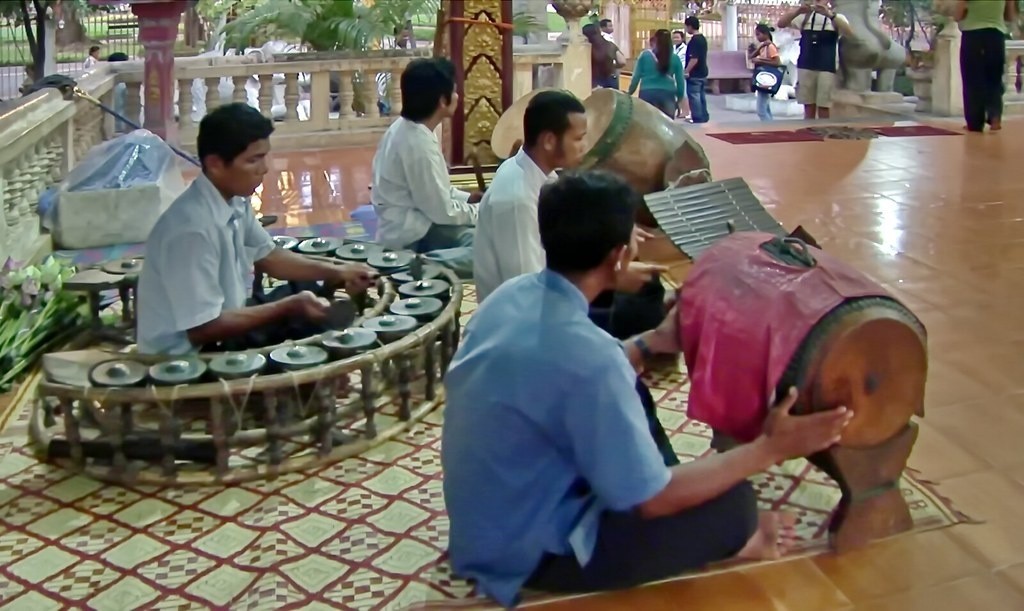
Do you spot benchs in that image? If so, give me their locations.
[708,51,754,95]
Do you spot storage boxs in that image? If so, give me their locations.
[59,155,175,251]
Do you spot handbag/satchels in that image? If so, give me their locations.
[751,43,788,97]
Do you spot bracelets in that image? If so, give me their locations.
[632,334,651,361]
[831,11,836,21]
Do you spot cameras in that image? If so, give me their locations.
[809,5,816,11]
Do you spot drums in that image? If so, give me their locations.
[568,87,710,229]
[89,234,452,388]
[676,231,926,449]
[490,88,576,158]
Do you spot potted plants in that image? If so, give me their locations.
[904,50,933,99]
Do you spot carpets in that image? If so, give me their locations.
[705,125,963,145]
[0,282,988,611]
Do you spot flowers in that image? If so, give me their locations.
[0,256,120,393]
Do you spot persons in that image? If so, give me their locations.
[582,16,709,124]
[748,24,780,121]
[472,89,670,341]
[440,169,855,608]
[138,107,381,397]
[954,0,1017,134]
[393,25,410,50]
[370,56,486,280]
[777,0,852,122]
[84,46,130,131]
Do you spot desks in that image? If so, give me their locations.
[29,236,463,484]
